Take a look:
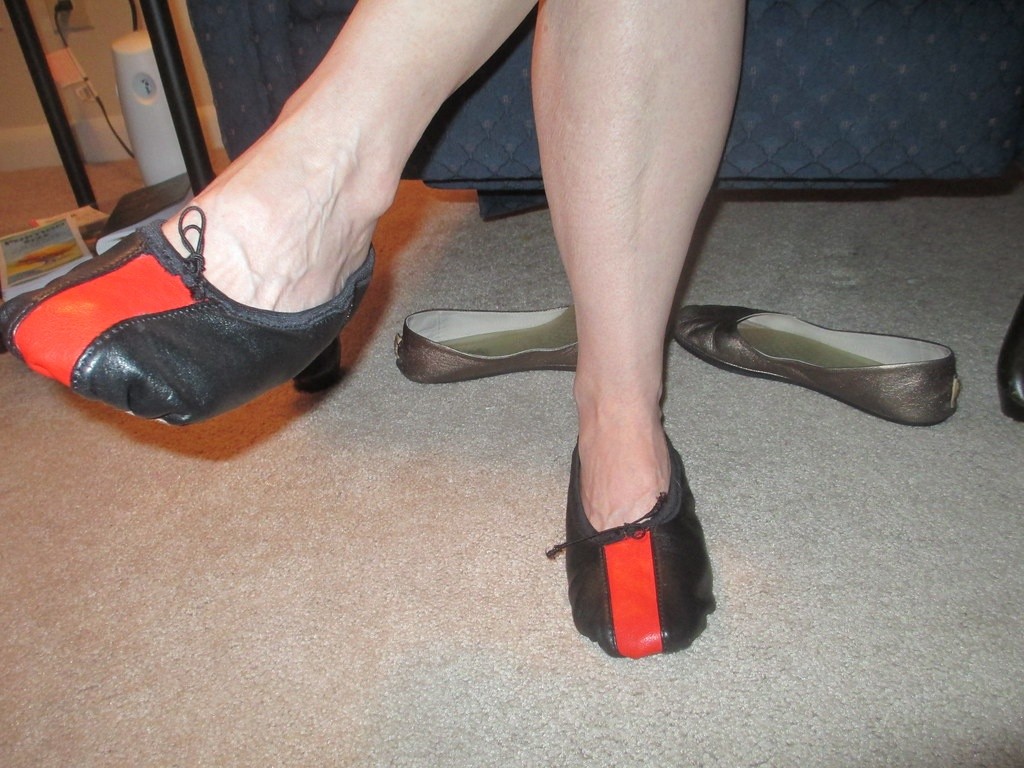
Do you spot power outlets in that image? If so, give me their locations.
[47,0,91,32]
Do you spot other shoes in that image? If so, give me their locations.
[393,303,584,384]
[549,418,714,660]
[0,215,374,427]
[671,302,960,426]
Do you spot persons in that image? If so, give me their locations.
[12,1,748,655]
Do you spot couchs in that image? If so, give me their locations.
[183,0,1024,230]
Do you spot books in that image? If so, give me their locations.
[0,217,93,302]
